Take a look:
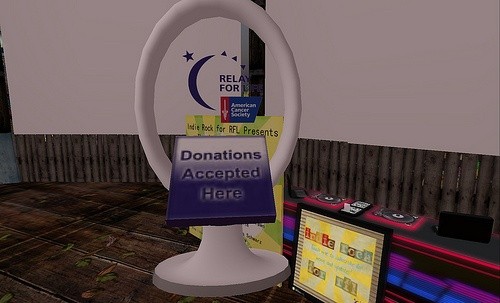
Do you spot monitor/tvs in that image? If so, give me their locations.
[290,202,392,302]
